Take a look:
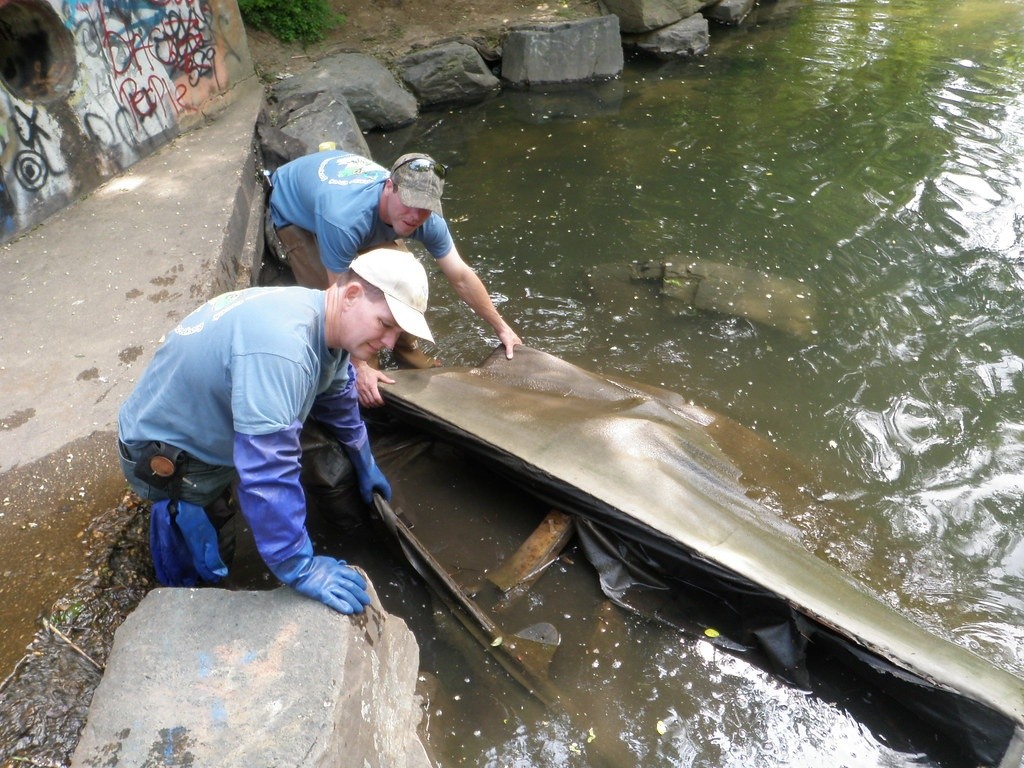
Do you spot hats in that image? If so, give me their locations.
[349,248,436,345]
[391,151,444,217]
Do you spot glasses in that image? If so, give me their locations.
[394,157,448,178]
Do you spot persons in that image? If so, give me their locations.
[266,150,523,408]
[117,248,436,615]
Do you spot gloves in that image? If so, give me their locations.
[232,425,371,614]
[313,361,393,504]
